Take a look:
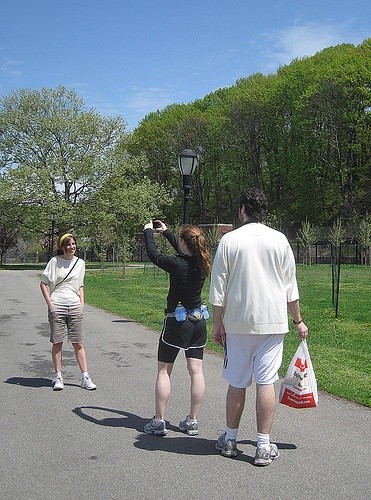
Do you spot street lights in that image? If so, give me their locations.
[177,149,198,224]
[48,211,57,262]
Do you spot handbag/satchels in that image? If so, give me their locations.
[279,336,318,409]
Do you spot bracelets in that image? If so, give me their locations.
[293,320,303,324]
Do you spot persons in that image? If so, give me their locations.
[210,187,309,465]
[143,220,210,436]
[40,233,97,391]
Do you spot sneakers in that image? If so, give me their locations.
[144,417,168,435]
[81,378,96,390]
[215,436,237,456]
[179,419,198,435]
[51,377,64,390]
[253,443,280,465]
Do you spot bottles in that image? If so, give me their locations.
[175,302,186,321]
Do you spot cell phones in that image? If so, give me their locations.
[153,222,161,228]
